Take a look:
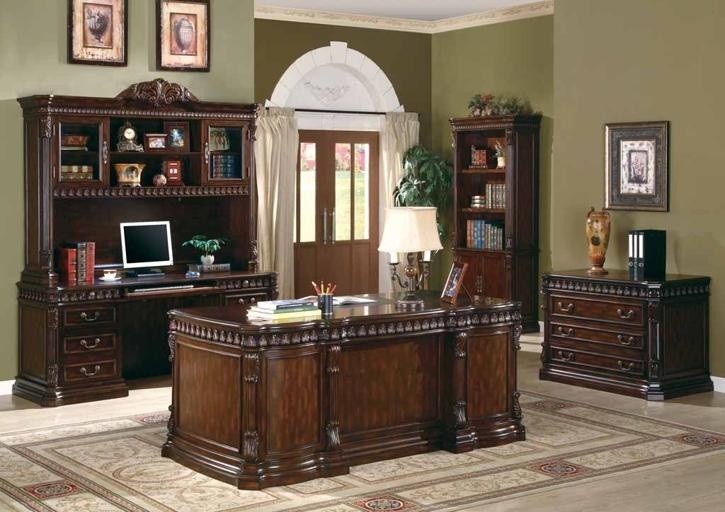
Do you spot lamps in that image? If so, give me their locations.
[377,206,443,306]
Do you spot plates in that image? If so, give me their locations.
[98,276,122,281]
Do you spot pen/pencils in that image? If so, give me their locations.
[312,279,337,295]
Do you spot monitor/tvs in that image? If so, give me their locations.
[119,220,174,278]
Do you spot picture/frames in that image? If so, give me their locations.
[143,132,169,153]
[165,121,190,152]
[67,0,128,67]
[439,260,473,303]
[208,127,231,151]
[157,0,211,72]
[603,121,671,212]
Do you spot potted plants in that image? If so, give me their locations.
[182,234,233,265]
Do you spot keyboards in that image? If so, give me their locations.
[128,285,194,293]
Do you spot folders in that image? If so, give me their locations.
[627,229,666,283]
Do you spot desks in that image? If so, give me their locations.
[161,291,526,489]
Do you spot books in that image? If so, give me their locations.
[465,182,507,252]
[236,154,243,178]
[57,248,77,281]
[212,154,221,178]
[468,144,491,172]
[61,165,94,173]
[228,154,235,177]
[86,241,94,281]
[61,172,93,180]
[187,263,230,273]
[64,240,86,282]
[220,154,228,178]
[300,295,377,306]
[246,298,323,325]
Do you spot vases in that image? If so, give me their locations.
[113,164,145,187]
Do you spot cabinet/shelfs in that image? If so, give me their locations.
[60,302,123,384]
[452,252,512,298]
[53,112,110,187]
[221,280,276,303]
[201,121,252,184]
[539,269,711,402]
[17,96,258,288]
[450,111,542,250]
[111,117,201,187]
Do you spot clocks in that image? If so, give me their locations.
[117,120,144,153]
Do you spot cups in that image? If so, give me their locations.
[102,269,117,278]
